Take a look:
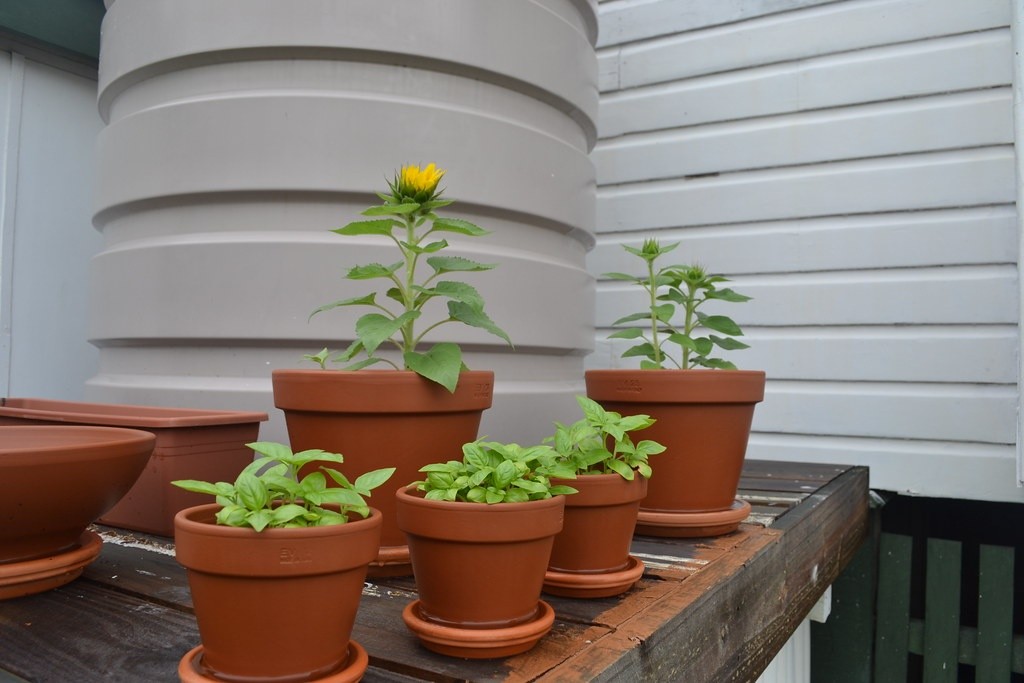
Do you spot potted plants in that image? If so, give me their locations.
[166,438,397,683]
[542,394,668,598]
[584,237,767,538]
[390,435,579,660]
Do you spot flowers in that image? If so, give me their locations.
[295,162,517,397]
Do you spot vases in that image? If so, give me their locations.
[273,370,495,577]
[0,422,159,604]
[0,396,269,537]
